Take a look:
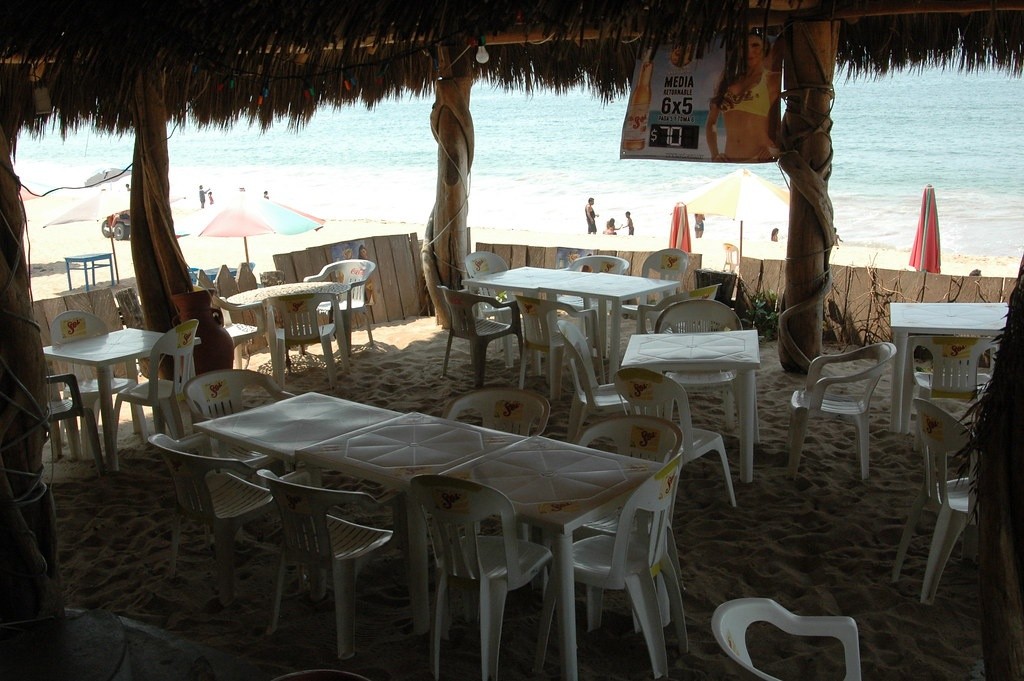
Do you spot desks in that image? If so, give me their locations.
[197,390,404,465]
[620,330,762,482]
[294,411,528,632]
[42,327,199,476]
[540,270,680,382]
[461,267,597,375]
[435,435,663,681]
[215,279,344,388]
[890,299,1007,426]
[65,252,115,291]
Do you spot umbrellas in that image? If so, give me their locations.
[170,191,326,272]
[909,183,943,276]
[668,201,692,253]
[660,167,791,278]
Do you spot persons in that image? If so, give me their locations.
[125,183,131,192]
[694,213,706,239]
[968,269,983,276]
[584,198,600,235]
[207,192,214,206]
[199,185,210,209]
[263,190,270,201]
[601,219,624,235]
[832,227,843,247]
[622,212,634,236]
[770,228,779,243]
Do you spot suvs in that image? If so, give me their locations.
[102,209,131,240]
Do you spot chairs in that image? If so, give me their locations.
[34,249,1015,681]
